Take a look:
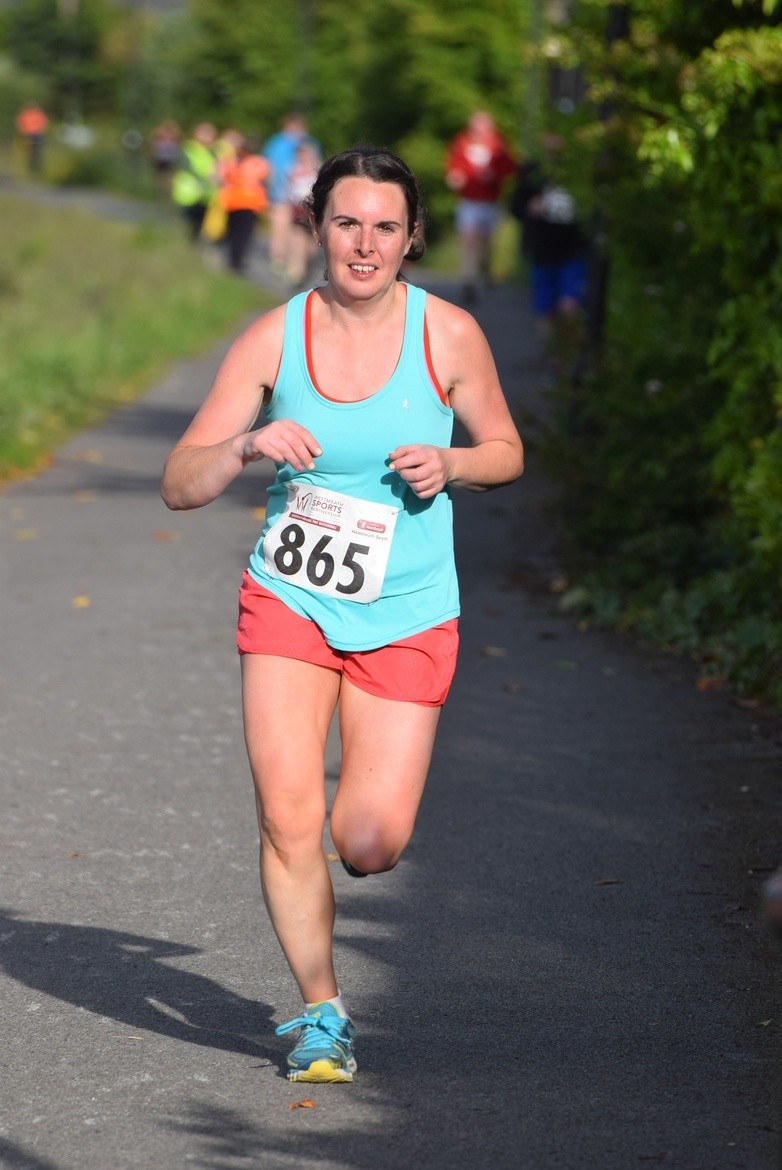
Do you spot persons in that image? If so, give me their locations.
[151,115,270,270]
[159,148,524,1082]
[511,128,606,386]
[261,112,326,284]
[17,96,49,171]
[445,113,519,300]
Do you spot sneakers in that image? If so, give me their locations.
[275,1001,357,1083]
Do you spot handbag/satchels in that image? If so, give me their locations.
[202,200,227,239]
